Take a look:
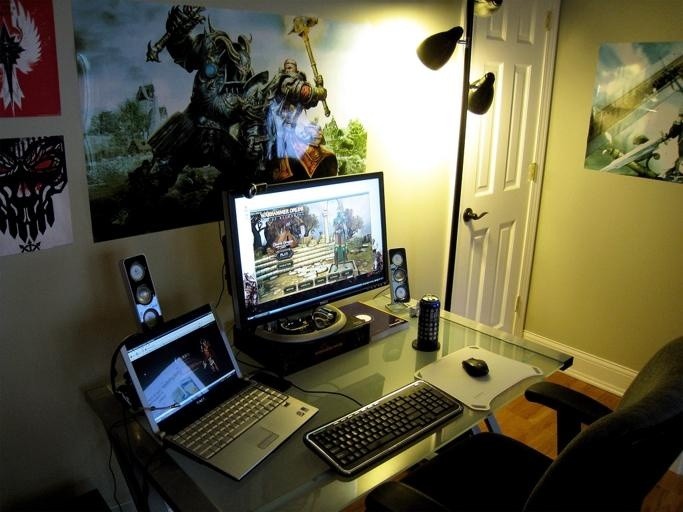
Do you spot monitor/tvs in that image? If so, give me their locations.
[222,170,390,343]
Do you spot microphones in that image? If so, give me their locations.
[136,403,181,411]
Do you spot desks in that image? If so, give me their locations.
[83,293,574,510]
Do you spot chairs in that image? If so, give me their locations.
[362,334,683,509]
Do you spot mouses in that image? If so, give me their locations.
[463,357,489,377]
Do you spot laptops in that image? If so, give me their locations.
[119,300,319,481]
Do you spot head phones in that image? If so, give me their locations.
[108,331,145,418]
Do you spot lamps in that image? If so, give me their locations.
[412,2,503,313]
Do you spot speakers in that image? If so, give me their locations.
[388,248,410,304]
[119,253,165,332]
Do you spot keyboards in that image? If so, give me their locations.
[303,380,464,474]
